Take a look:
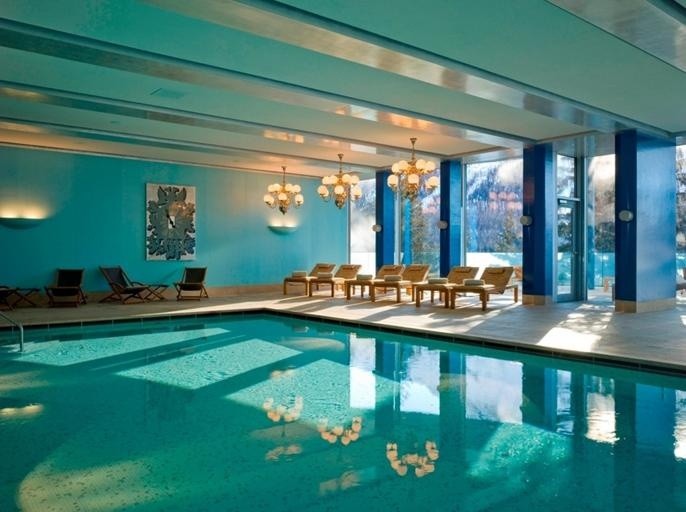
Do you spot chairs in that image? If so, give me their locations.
[283,259,518,312]
[44,268,91,307]
[98,264,147,305]
[174,267,209,302]
[0,275,42,311]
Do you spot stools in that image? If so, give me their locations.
[144,283,168,301]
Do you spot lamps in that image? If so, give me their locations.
[261,165,303,215]
[263,368,440,485]
[315,152,361,208]
[386,137,437,200]
